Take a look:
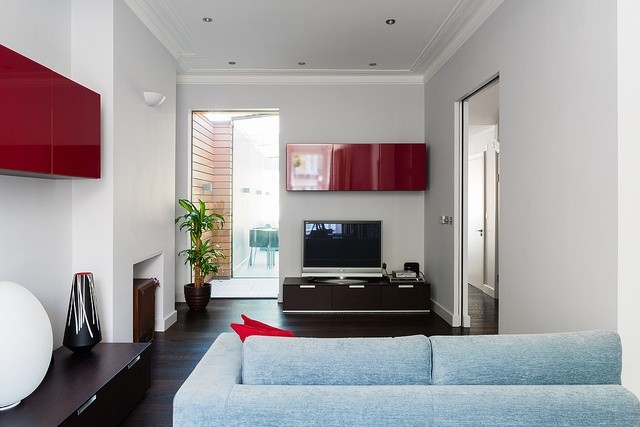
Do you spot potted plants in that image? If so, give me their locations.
[175,199,227,314]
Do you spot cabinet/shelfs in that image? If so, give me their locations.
[52,70,101,178]
[133,279,157,343]
[0,44,52,175]
[286,144,332,191]
[380,143,426,191]
[333,143,380,191]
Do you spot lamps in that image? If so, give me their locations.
[143,90,166,108]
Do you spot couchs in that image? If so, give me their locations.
[172,331,640,425]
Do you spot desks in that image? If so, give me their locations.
[0,342,152,426]
[283,277,429,313]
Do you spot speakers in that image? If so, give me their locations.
[403,261,419,275]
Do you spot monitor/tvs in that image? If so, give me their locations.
[301,219,382,285]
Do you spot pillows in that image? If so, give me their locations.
[229,323,291,346]
[241,315,287,332]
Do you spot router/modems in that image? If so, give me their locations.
[393,271,417,277]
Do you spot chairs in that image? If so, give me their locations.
[268,226,279,266]
[249,226,269,266]
